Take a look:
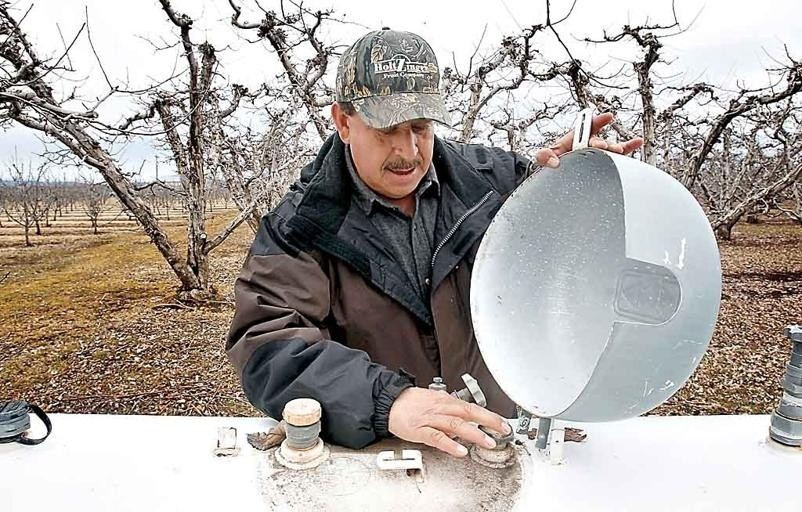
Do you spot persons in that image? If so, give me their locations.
[224,27,649,458]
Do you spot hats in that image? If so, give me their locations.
[336,27,452,129]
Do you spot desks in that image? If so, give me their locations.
[1,414,802,512]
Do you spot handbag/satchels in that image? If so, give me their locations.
[1,401,30,443]
